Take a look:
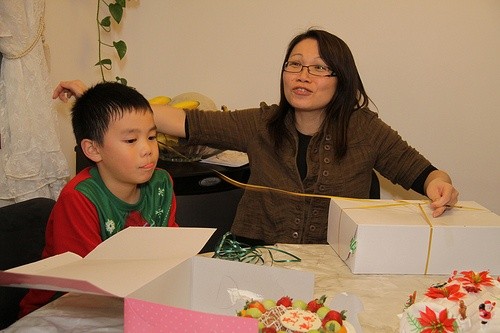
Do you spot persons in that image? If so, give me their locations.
[18,82,180,318]
[52,29,459,245]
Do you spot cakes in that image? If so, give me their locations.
[233,294,355,333]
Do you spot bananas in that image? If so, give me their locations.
[147,96,200,109]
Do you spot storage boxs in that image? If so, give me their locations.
[0,223,316,333]
[327,195,500,275]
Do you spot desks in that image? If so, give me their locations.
[0,244,500,333]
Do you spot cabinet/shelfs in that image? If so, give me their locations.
[76,144,251,254]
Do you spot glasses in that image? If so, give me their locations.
[284,61,337,77]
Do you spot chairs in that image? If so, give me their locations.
[0,197,57,331]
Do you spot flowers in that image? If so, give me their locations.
[425,270,500,302]
[404,291,417,309]
[407,306,460,333]
